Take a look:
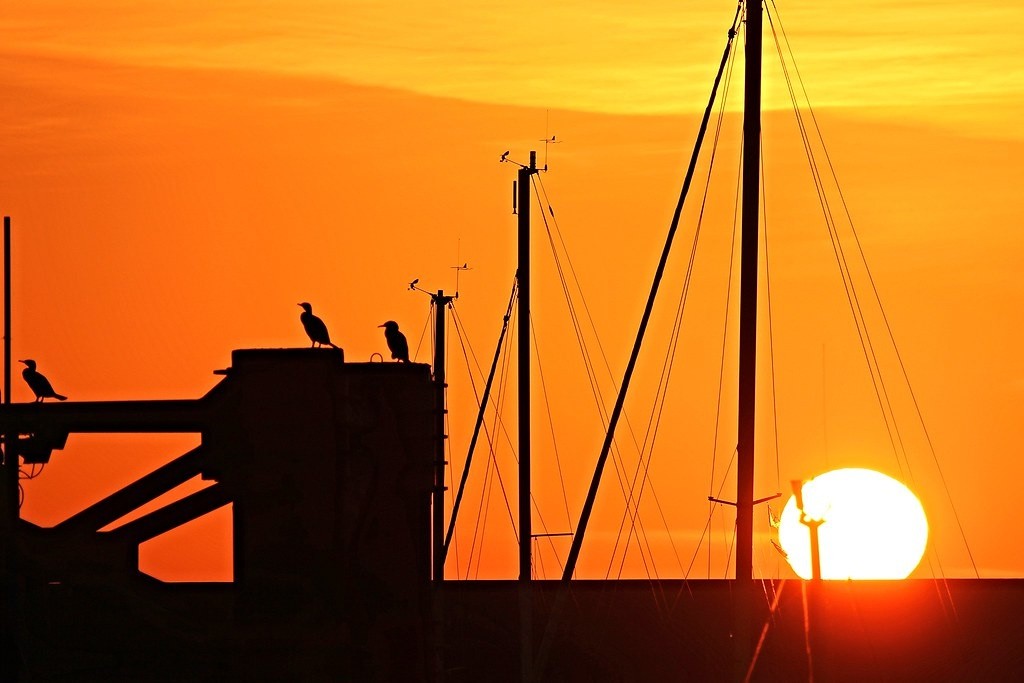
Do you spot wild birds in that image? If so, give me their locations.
[297,302,339,348]
[19,360,67,402]
[378,320,412,365]
